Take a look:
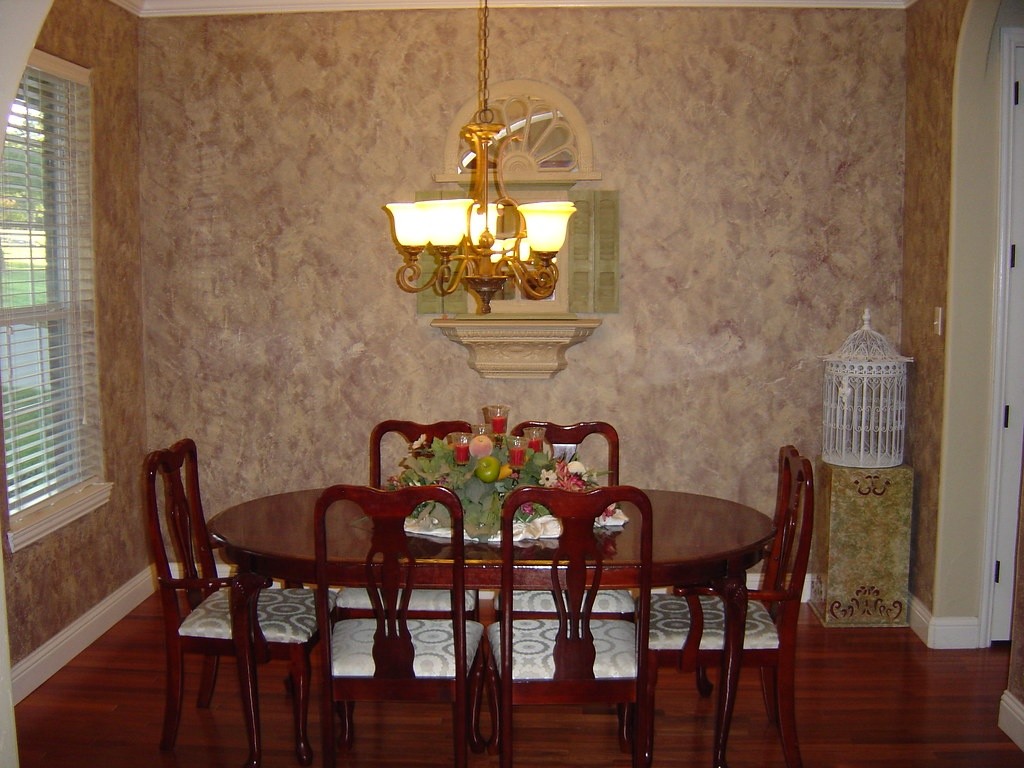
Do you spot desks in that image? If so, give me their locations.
[205,485,777,768]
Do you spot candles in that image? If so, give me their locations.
[492,415,509,434]
[527,436,544,452]
[453,442,469,464]
[508,447,525,466]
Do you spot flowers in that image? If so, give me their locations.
[381,433,619,544]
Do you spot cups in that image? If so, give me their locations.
[453,432,472,464]
[483,406,508,434]
[473,424,493,442]
[524,428,546,451]
[506,436,530,467]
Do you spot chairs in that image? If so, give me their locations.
[140,420,814,768]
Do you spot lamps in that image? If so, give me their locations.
[380,2,579,316]
